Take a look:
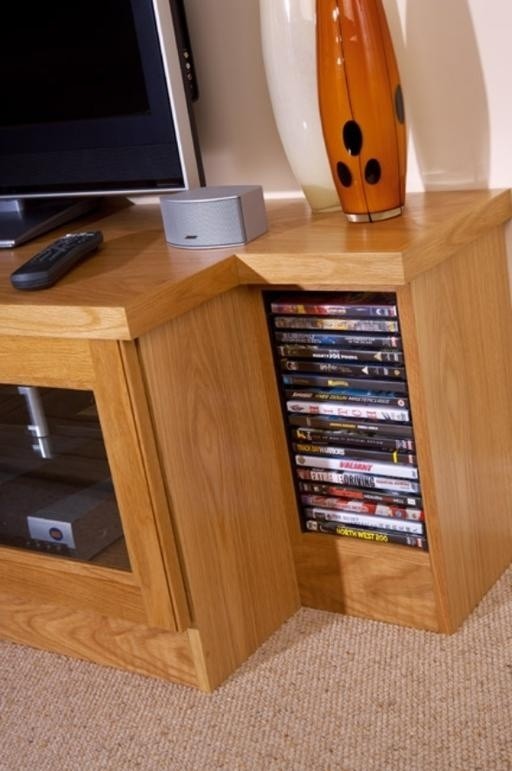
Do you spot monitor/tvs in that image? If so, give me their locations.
[0,0,207,249]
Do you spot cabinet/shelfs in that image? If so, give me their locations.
[0,188,512,695]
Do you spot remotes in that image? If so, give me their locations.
[11,230,103,290]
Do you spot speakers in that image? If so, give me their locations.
[159,184,268,248]
